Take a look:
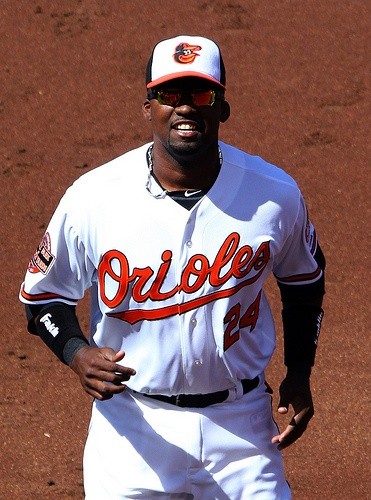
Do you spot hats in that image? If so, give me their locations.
[145,34,226,91]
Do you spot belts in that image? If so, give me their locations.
[130,375,259,408]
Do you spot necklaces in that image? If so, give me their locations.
[146,142,223,199]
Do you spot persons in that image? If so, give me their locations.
[19,35,326,500]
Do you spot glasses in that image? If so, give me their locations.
[147,85,224,110]
